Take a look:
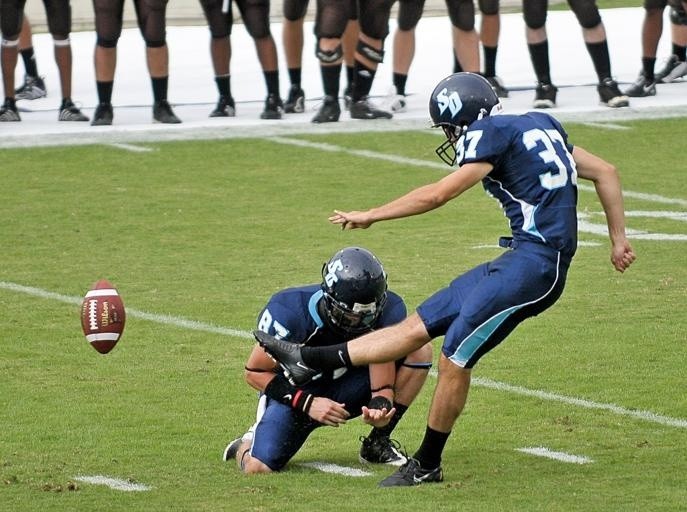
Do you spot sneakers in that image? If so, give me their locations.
[357,434,411,468]
[15,74,46,101]
[222,425,253,462]
[596,77,630,108]
[90,102,113,125]
[486,75,509,98]
[153,98,181,123]
[654,53,687,84]
[378,456,444,488]
[624,71,656,97]
[58,101,88,121]
[532,82,558,109]
[259,89,407,124]
[209,94,236,117]
[0,104,21,121]
[251,330,323,391]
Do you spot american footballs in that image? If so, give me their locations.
[80,278,126,354]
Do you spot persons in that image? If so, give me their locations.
[220,249,433,465]
[93,2,180,126]
[255,72,634,488]
[523,2,630,109]
[313,2,393,120]
[200,2,284,119]
[2,2,90,120]
[625,2,685,97]
[391,2,508,113]
[283,2,359,113]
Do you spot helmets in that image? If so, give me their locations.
[428,73,505,168]
[320,246,388,334]
